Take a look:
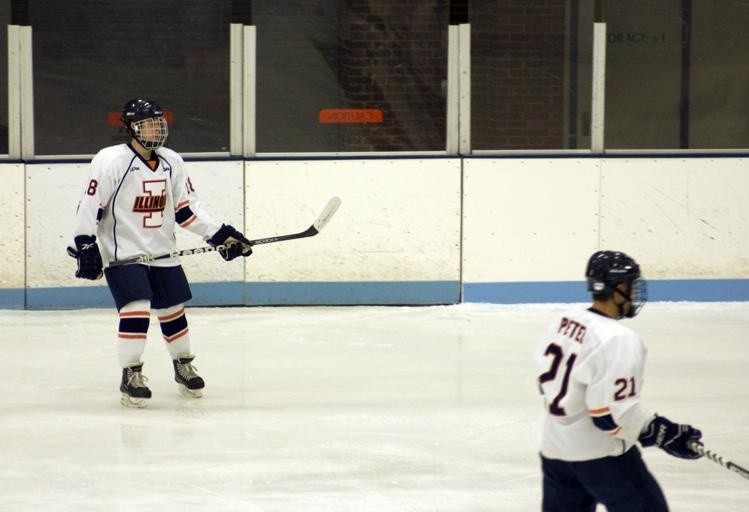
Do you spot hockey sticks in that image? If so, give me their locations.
[102,196,345,267]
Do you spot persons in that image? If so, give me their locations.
[528,249,704,510]
[66,98,253,406]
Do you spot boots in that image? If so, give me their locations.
[173,354,205,389]
[120,362,152,397]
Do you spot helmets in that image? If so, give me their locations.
[584,251,649,318]
[121,98,168,152]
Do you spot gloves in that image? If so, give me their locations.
[638,415,705,459]
[74,235,103,279]
[207,226,253,261]
